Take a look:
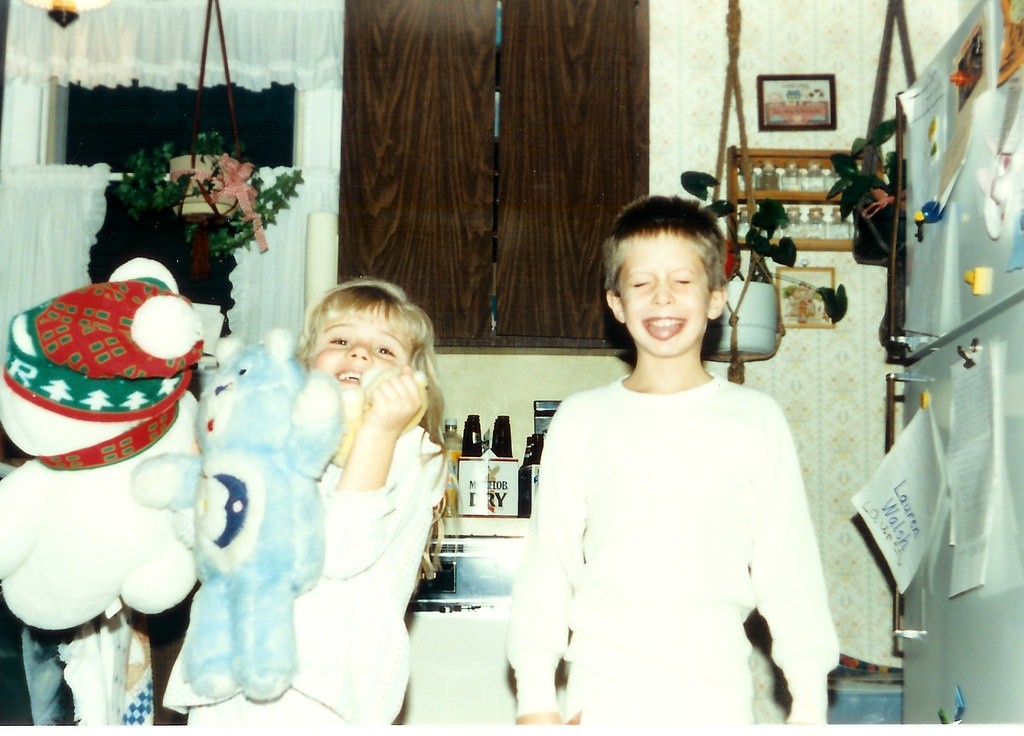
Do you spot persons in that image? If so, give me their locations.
[161,279,460,725]
[507,195,842,725]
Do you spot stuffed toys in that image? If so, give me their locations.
[0,256,429,704]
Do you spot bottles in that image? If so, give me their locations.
[518,437,532,517]
[492,415,514,458]
[524,433,544,518]
[850,221,859,238]
[779,160,803,191]
[440,418,462,518]
[801,160,826,191]
[737,162,757,191]
[783,207,806,238]
[756,158,780,191]
[826,207,850,239]
[737,208,751,238]
[804,208,827,238]
[760,226,781,238]
[463,414,482,458]
[825,164,841,191]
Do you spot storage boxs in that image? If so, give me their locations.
[458,457,519,516]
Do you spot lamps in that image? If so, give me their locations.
[22,0,111,27]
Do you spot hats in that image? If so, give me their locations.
[4,277,205,422]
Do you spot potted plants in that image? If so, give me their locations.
[681,171,848,354]
[112,128,305,257]
[826,119,907,259]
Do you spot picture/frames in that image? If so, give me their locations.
[776,266,836,329]
[757,74,836,131]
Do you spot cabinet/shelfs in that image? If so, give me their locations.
[727,146,885,250]
